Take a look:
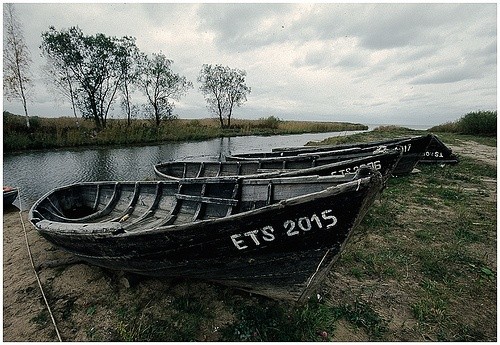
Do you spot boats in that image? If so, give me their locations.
[28,168,384,305]
[225,134,434,177]
[272,132,458,166]
[154,145,404,192]
